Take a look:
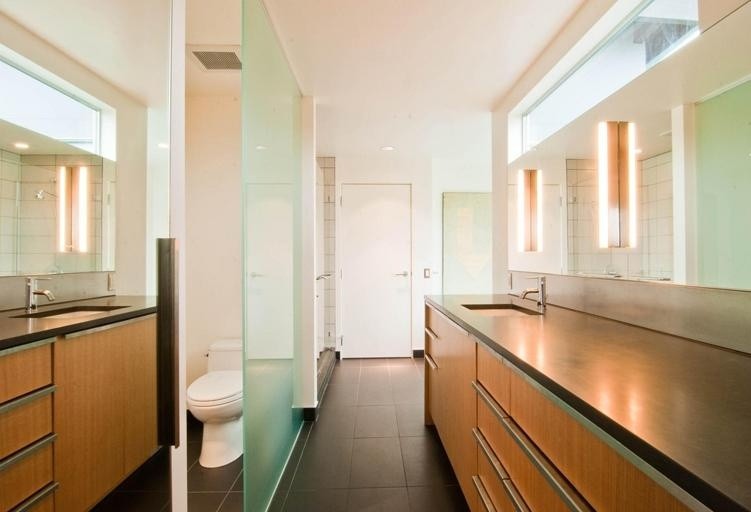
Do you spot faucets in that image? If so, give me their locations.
[24,276,56,310]
[520,275,547,307]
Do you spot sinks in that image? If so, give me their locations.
[460,303,544,317]
[8,304,132,320]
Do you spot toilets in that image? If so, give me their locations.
[186,339,244,470]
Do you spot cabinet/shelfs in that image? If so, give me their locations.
[54,307,172,512]
[476,327,742,512]
[0,331,59,512]
[419,295,477,512]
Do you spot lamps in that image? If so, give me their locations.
[531,168,543,252]
[594,119,620,249]
[70,165,92,255]
[619,119,638,249]
[51,162,70,252]
[516,169,530,252]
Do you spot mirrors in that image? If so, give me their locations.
[0,0,176,512]
[0,44,119,279]
[502,1,750,294]
